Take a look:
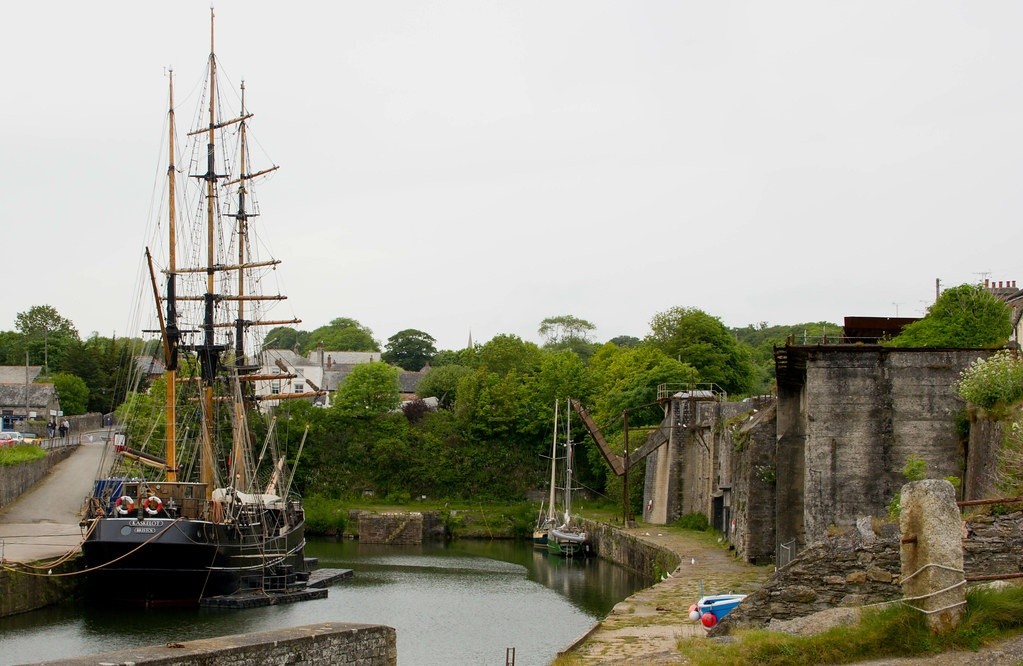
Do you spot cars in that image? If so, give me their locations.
[0,433,42,450]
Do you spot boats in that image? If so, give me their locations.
[698,594,749,633]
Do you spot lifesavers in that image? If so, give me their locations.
[115,495,135,515]
[143,495,163,515]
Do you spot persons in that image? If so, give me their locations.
[47,420,57,439]
[58,419,70,438]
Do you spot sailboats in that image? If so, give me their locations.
[532,399,560,545]
[545,396,591,556]
[73,7,307,614]
